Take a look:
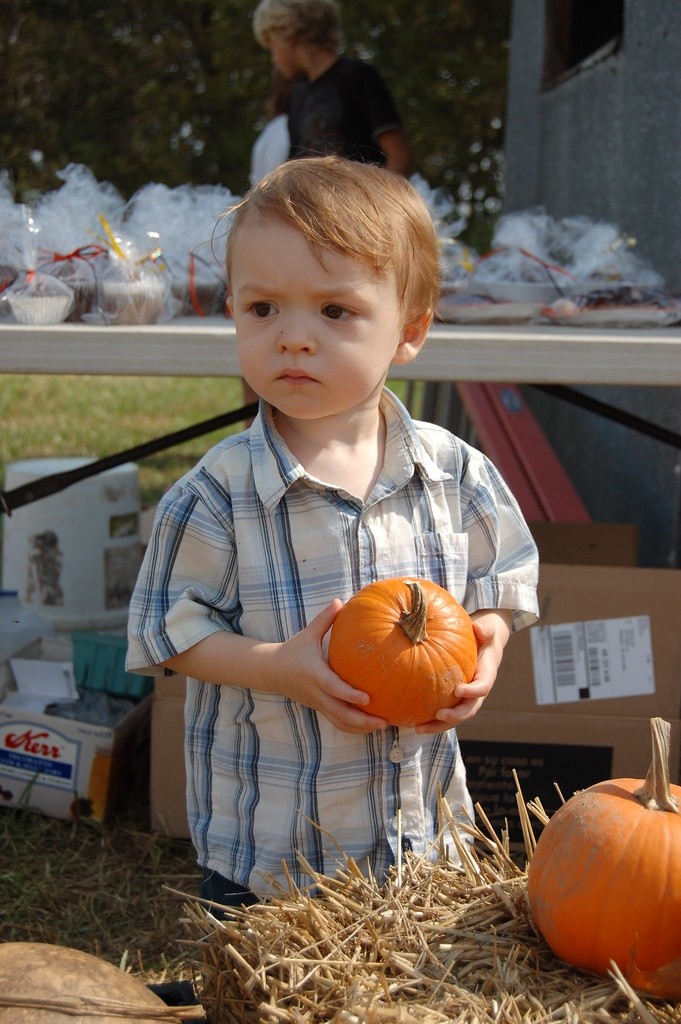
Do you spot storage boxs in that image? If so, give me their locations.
[0,596,188,839]
[450,521,681,868]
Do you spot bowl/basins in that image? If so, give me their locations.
[171,280,220,316]
[8,297,71,323]
[106,282,164,326]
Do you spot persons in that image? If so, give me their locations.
[126,1,540,922]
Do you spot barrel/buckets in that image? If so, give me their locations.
[1,456,141,624]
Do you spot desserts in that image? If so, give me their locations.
[0,256,229,326]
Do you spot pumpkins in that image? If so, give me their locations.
[327,577,478,726]
[529,716,681,1002]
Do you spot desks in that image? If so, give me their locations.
[0,313,681,519]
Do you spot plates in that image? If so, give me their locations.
[436,304,542,323]
[545,310,679,329]
[473,279,570,302]
[562,279,666,301]
